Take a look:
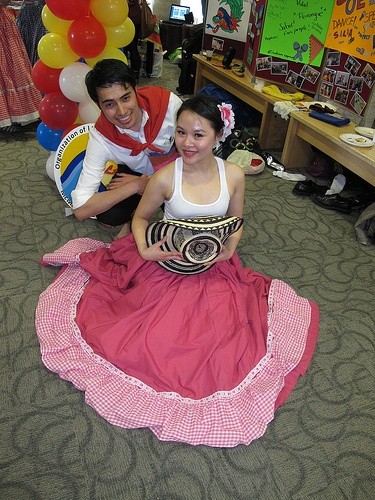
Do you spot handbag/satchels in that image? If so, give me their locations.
[139,0,157,40]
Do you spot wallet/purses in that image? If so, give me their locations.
[308,110,350,127]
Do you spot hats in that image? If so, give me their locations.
[145,215,245,275]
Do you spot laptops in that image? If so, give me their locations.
[162,4,190,25]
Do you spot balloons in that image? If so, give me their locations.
[31,0,135,182]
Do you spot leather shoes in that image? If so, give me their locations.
[311,189,353,215]
[293,179,330,197]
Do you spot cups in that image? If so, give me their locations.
[206,50,213,62]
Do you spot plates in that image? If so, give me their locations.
[339,133,374,147]
[354,126,375,138]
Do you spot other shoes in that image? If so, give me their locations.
[132,70,140,79]
[0,125,16,134]
[260,151,285,171]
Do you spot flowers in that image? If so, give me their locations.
[216,102,235,142]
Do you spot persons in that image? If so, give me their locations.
[70,58,221,242]
[35,96,320,449]
[117,0,157,87]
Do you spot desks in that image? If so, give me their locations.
[193,54,312,150]
[281,111,375,186]
[160,23,183,59]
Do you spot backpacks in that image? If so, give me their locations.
[175,35,202,95]
